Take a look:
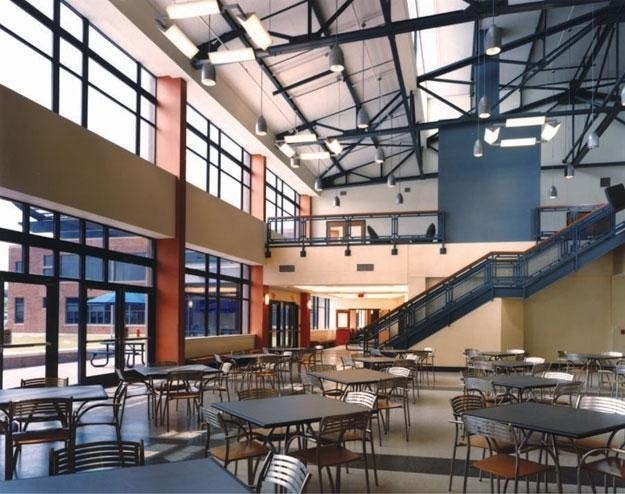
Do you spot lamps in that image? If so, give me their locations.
[266,241,446,257]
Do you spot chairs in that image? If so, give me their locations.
[368,225,379,244]
[422,224,434,242]
